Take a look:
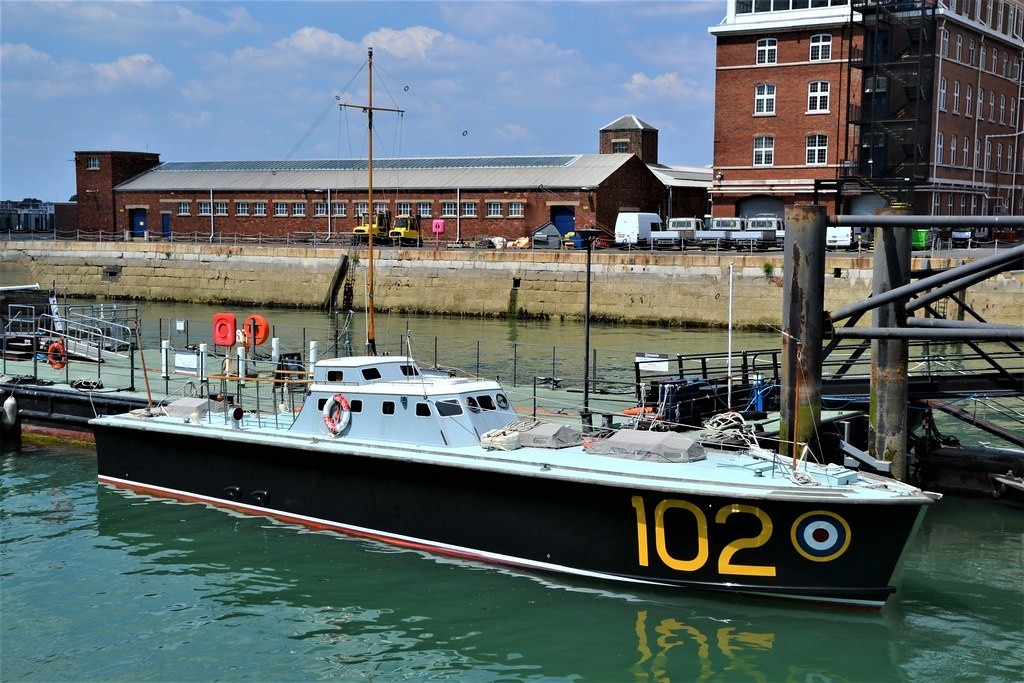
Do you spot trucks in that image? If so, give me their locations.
[650,216,709,251]
[695,215,785,251]
[951,226,988,247]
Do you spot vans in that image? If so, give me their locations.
[613,211,666,247]
[826,227,873,252]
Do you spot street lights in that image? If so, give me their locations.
[574,227,605,413]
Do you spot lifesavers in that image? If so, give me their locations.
[244,315,270,345]
[624,406,653,415]
[323,393,351,433]
[47,343,65,369]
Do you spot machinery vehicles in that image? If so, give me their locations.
[389,214,423,249]
[350,210,392,247]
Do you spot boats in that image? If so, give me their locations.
[85,44,945,609]
[0,279,66,360]
